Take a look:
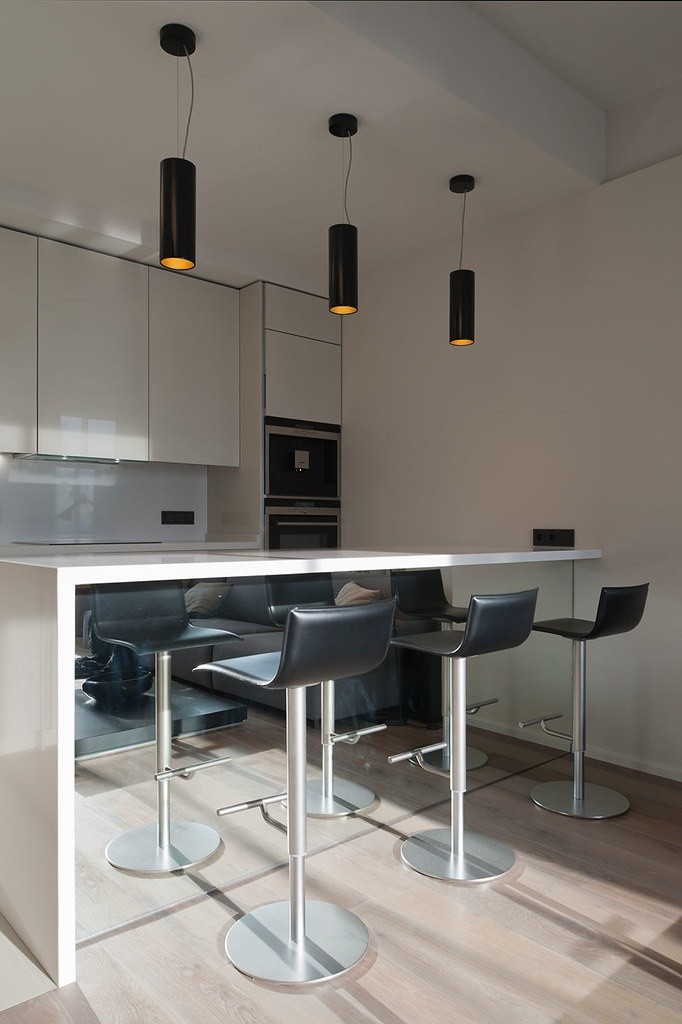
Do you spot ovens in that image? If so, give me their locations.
[264,498,340,550]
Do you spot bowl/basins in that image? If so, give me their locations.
[82,669,152,702]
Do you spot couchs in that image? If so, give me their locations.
[75,570,403,729]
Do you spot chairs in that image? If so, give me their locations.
[391,587,541,883]
[90,579,244,874]
[531,584,651,820]
[193,596,398,990]
[265,572,387,818]
[391,570,498,773]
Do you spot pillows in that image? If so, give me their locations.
[185,583,233,619]
[334,580,382,607]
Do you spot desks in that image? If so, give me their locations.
[0,544,602,991]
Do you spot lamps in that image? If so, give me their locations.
[449,175,474,346]
[159,23,196,270]
[328,113,358,315]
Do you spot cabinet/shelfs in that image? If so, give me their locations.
[0,225,342,467]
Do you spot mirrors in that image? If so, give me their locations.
[80,563,578,947]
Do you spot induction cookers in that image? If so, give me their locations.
[13,539,163,545]
